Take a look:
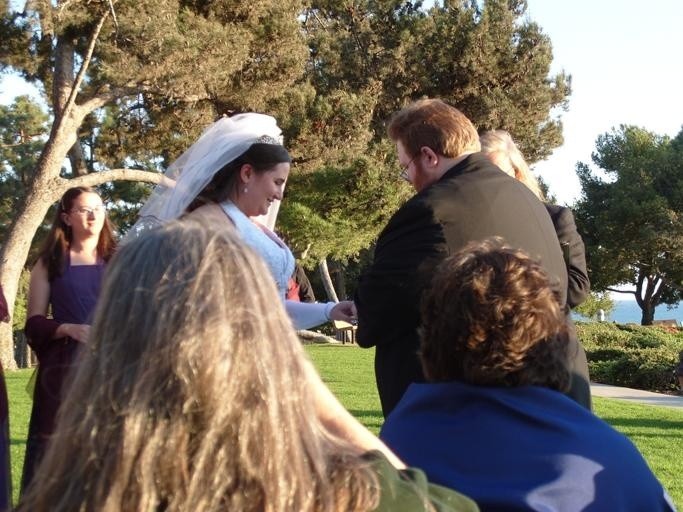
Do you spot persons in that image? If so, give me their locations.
[352,97,594,420]
[374,235,676,512]
[8,218,481,512]
[113,111,359,332]
[18,186,126,500]
[478,128,591,410]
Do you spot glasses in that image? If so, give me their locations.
[399,156,417,184]
[70,205,106,216]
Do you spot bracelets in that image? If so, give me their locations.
[322,300,334,321]
